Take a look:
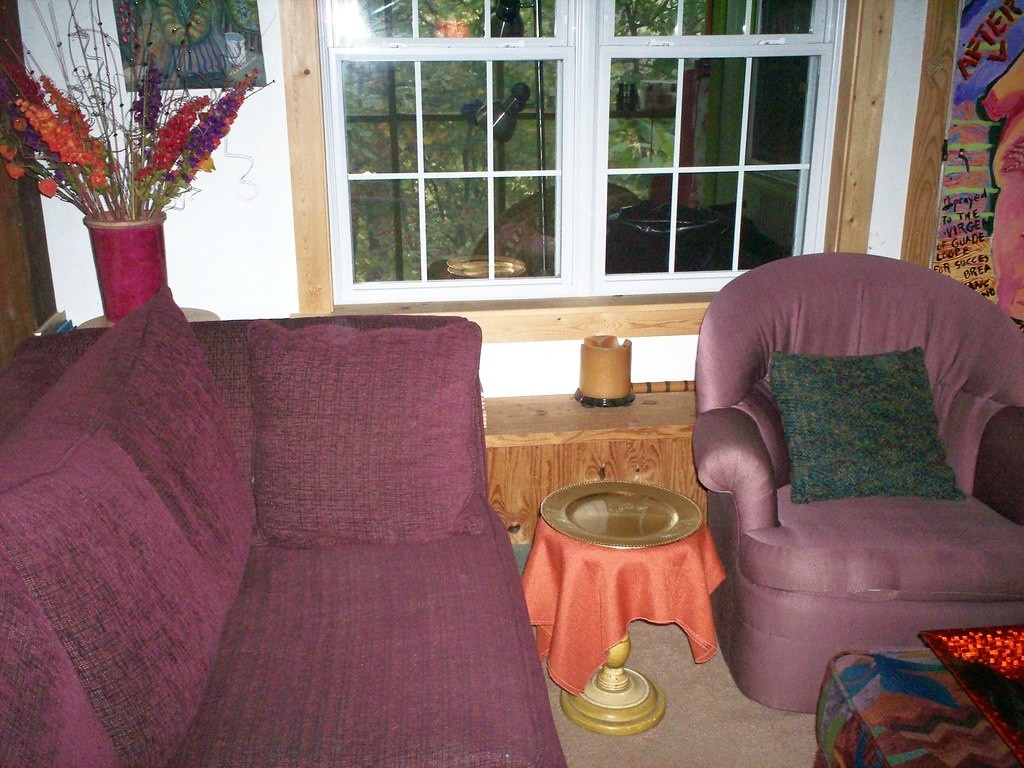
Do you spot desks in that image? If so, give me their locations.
[523,514,726,735]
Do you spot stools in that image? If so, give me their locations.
[814,652,1024,768]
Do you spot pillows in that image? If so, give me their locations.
[770,347,967,504]
[247,319,492,550]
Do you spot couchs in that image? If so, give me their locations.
[0,279,568,768]
[691,252,1024,714]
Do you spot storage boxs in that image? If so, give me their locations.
[640,81,677,110]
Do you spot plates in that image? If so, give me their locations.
[541,479,703,550]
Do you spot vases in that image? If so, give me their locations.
[84,210,168,322]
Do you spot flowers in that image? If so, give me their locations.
[0,1,277,222]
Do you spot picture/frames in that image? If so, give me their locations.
[744,0,813,173]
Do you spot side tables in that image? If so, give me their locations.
[80,307,220,328]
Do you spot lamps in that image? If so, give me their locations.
[479,0,525,38]
[476,83,531,143]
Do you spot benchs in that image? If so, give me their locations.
[482,390,710,545]
